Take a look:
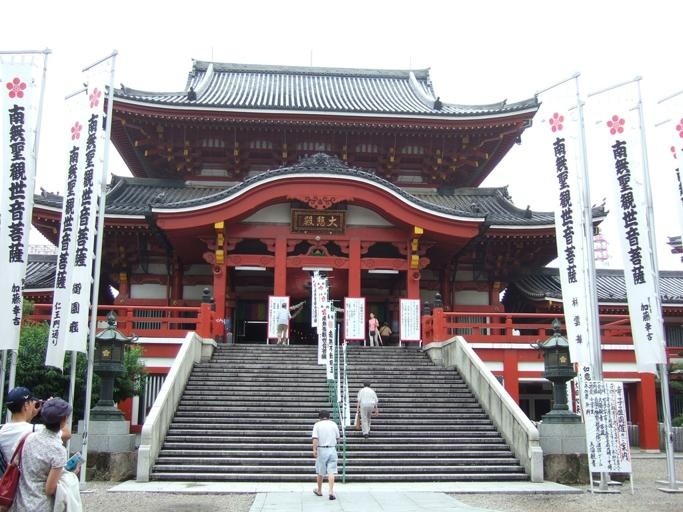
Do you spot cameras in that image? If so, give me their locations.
[35,401,46,409]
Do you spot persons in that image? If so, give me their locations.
[275,303,290,345]
[356,381,378,438]
[378,322,392,346]
[0,387,72,512]
[27,396,55,422]
[311,410,341,499]
[367,312,380,346]
[7,398,81,512]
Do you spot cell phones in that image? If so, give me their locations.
[65,452,84,471]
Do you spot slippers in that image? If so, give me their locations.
[312,488,336,499]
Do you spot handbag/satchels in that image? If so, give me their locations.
[370,330,375,335]
[353,410,360,430]
[0,430,33,508]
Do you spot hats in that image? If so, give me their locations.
[4,386,39,405]
[39,395,74,424]
